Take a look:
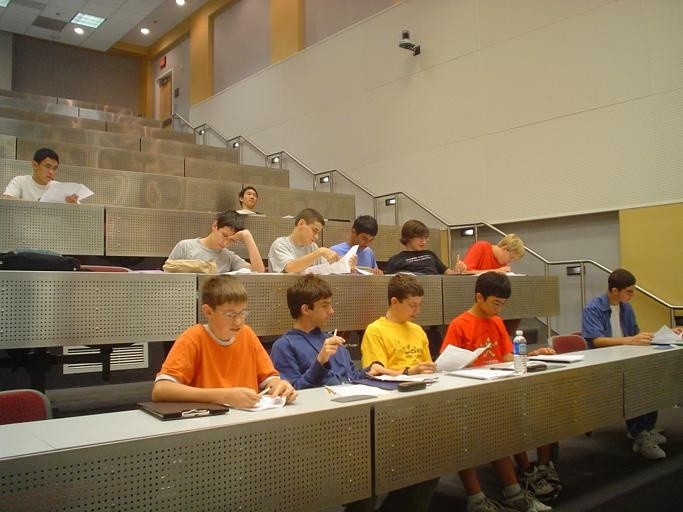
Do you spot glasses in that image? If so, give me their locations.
[217,311,249,318]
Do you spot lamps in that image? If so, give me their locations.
[398,29,420,56]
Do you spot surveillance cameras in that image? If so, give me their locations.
[398,30,418,52]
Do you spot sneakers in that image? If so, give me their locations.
[466,459,562,512]
[628,430,666,459]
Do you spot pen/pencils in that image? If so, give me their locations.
[334,329,337,337]
[258,386,272,396]
[490,368,515,371]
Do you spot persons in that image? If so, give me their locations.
[459,230,526,273]
[238,185,264,216]
[382,218,467,276]
[438,269,564,504]
[580,268,683,461]
[269,273,440,512]
[151,270,299,409]
[266,206,359,275]
[0,145,81,204]
[162,208,267,275]
[360,270,556,512]
[330,214,384,276]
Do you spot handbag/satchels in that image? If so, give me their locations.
[163,259,216,273]
[0,251,80,271]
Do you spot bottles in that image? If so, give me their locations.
[512,330,527,375]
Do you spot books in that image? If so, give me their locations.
[140,324,682,421]
[220,266,254,274]
[40,179,94,202]
[303,242,359,277]
[355,264,374,276]
